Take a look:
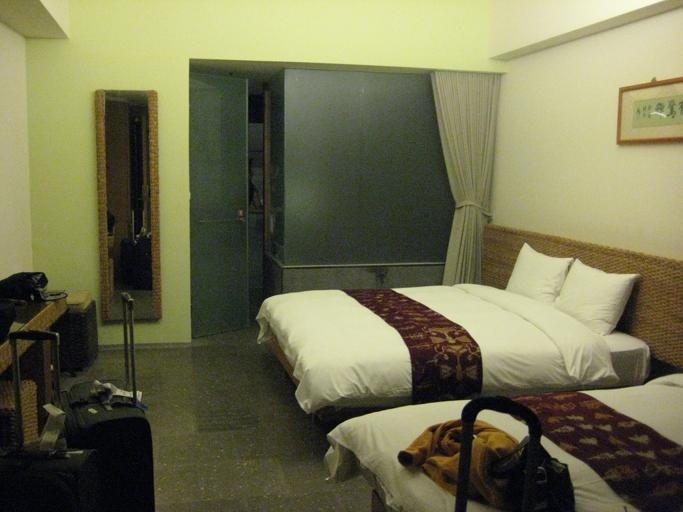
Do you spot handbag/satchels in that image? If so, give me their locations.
[506,438,575,512]
[0,271,48,300]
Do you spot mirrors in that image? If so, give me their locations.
[95,87,164,323]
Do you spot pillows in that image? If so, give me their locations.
[503,241,641,339]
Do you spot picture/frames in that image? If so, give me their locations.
[616,75,683,144]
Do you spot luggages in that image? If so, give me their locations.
[0,332,99,512]
[57,292,156,512]
[120,198,152,290]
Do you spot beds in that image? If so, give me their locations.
[325,373,683,512]
[257,283,652,433]
